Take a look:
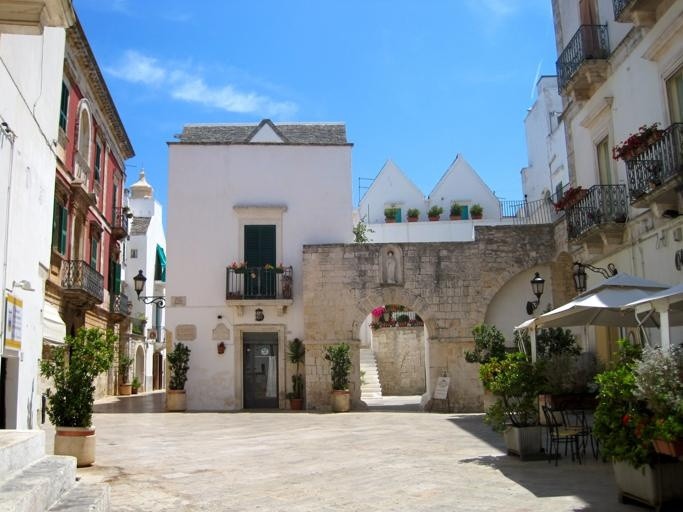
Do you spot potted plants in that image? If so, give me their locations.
[476,350,546,463]
[320,342,354,413]
[469,203,482,219]
[592,333,683,512]
[383,207,398,223]
[164,343,191,412]
[36,328,119,467]
[448,204,462,220]
[117,351,140,396]
[427,206,443,221]
[406,208,420,222]
[284,337,306,409]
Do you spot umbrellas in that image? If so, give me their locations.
[618,285,682,319]
[510,270,682,328]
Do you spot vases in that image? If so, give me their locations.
[621,131,661,162]
[562,189,587,211]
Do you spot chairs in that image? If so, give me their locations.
[540,404,610,469]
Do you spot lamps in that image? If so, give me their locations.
[526,272,545,313]
[572,262,617,292]
[131,269,166,308]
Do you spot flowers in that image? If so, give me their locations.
[549,186,582,213]
[609,120,658,161]
